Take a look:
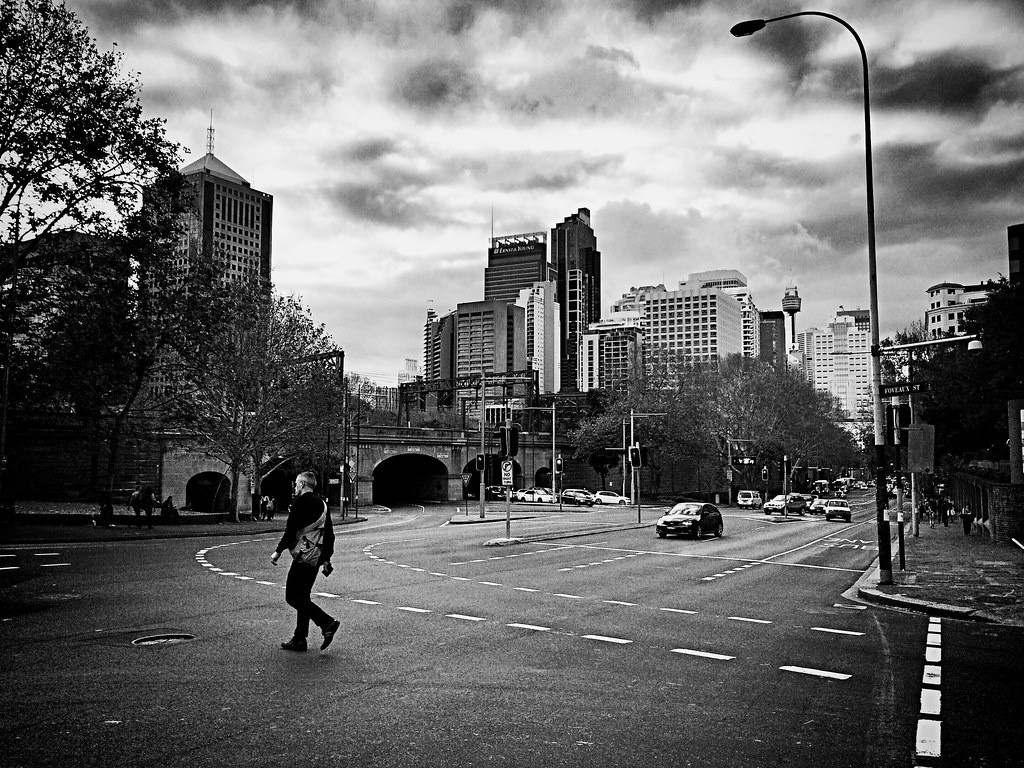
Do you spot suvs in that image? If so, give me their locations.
[764,494,808,517]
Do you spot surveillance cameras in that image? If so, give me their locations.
[968,341,983,355]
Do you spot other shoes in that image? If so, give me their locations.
[92,520,96,526]
[109,524,115,527]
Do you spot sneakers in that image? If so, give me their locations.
[281,639,307,652]
[321,620,340,650]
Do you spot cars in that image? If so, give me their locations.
[561,488,596,507]
[485,485,560,503]
[823,498,852,523]
[594,490,631,506]
[801,474,910,515]
[654,501,724,540]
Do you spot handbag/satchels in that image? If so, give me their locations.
[289,511,326,567]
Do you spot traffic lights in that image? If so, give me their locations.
[508,427,520,457]
[761,469,769,482]
[630,447,641,468]
[493,425,508,458]
[555,457,563,473]
[475,452,485,471]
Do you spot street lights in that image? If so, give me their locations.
[727,9,895,585]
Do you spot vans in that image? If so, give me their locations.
[738,489,762,510]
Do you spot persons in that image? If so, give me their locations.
[925,497,951,529]
[270,472,340,651]
[962,504,972,535]
[161,496,179,517]
[128,487,157,529]
[252,488,276,522]
